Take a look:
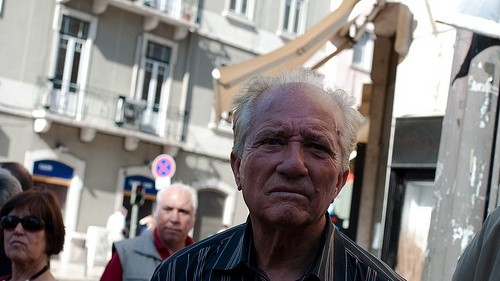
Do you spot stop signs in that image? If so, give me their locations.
[150,154,177,180]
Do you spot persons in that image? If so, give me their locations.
[452,205,500,281]
[0,161,198,281]
[150,69,407,281]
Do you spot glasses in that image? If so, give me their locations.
[0,215,47,232]
